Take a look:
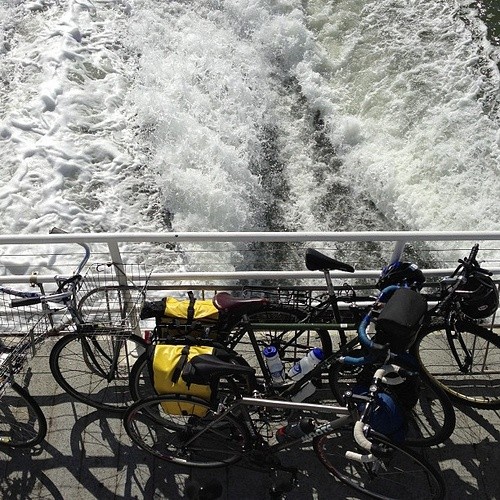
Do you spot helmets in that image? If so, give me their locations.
[462,273,499,318]
[378,261,426,290]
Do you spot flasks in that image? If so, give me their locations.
[275,417,316,444]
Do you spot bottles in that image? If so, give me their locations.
[288,378,319,404]
[263,345,286,384]
[289,348,322,381]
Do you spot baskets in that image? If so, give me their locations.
[1,288,55,399]
[58,263,153,340]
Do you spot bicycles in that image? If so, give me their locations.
[1,227,500,500]
